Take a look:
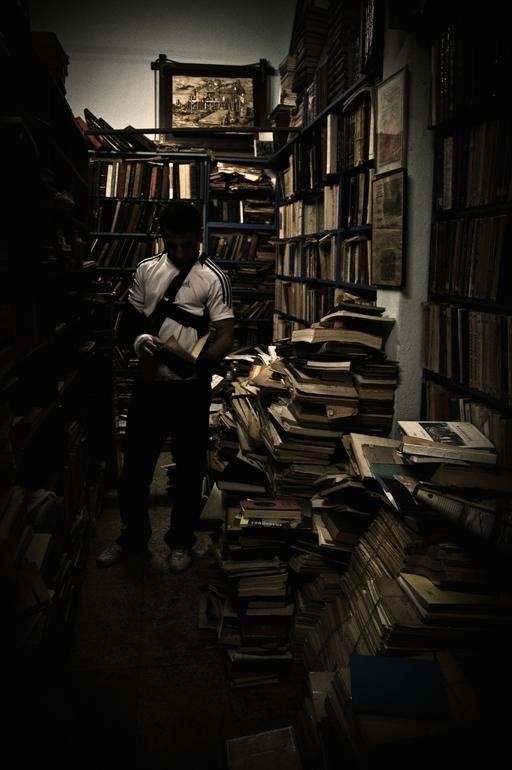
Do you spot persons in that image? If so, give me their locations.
[95,201,235,569]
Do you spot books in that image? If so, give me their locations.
[79,108,206,436]
[2,27,117,770]
[208,2,512,770]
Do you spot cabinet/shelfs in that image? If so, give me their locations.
[0,0,512,708]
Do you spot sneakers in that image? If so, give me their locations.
[167,546,191,574]
[96,540,127,566]
